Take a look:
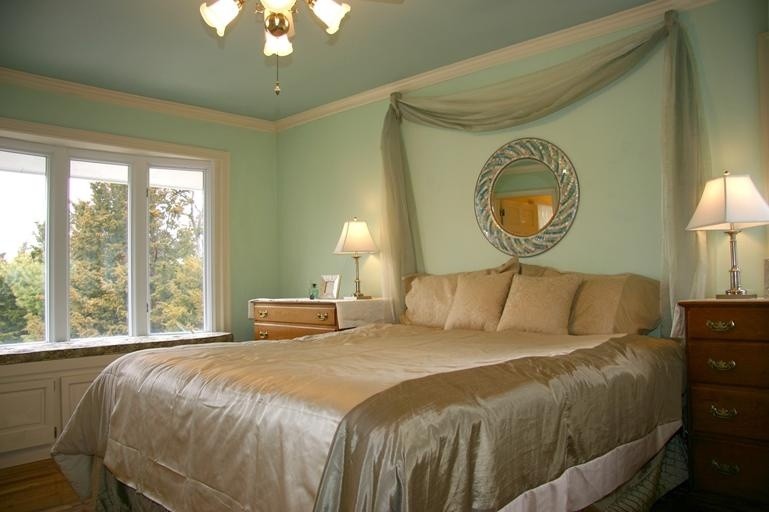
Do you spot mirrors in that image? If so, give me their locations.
[473,137,579,258]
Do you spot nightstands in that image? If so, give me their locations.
[248,299,394,342]
[677,298,766,505]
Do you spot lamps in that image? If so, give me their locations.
[334,216,381,298]
[687,170,769,298]
[198,0,351,96]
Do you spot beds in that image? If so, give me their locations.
[50,257,711,512]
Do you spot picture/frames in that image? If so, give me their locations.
[319,273,341,299]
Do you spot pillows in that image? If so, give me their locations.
[518,262,671,338]
[400,253,518,328]
[495,276,584,335]
[405,273,461,326]
[444,271,511,332]
